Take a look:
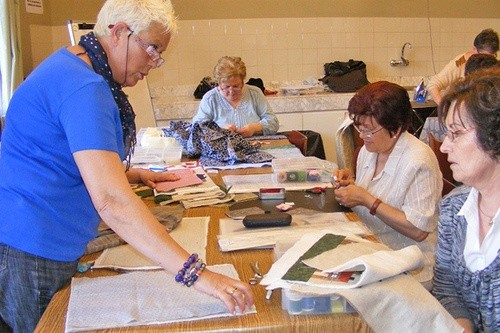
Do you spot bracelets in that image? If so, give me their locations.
[175,252,207,287]
[369,198,382,215]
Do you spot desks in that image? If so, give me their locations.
[410,101,438,135]
[34,135,434,333]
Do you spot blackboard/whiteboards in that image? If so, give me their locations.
[68,20,157,131]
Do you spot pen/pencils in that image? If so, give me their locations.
[262,285,275,301]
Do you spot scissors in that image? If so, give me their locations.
[248,260,268,287]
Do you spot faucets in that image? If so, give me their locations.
[401,42,412,60]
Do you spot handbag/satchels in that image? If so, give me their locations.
[318,58,371,93]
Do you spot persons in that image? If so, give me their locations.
[192,56,279,139]
[332,81,444,292]
[430,65,500,333]
[0,0,256,333]
[428,29,500,112]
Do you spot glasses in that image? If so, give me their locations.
[221,82,242,91]
[443,128,478,138]
[107,23,166,68]
[354,124,384,138]
